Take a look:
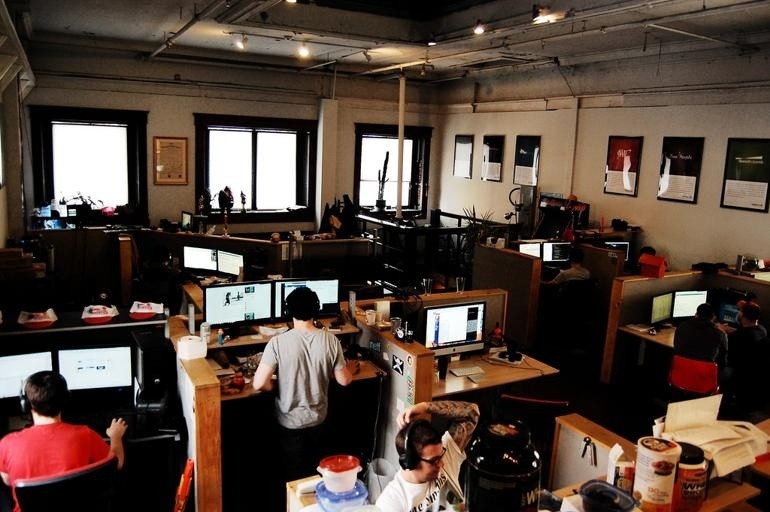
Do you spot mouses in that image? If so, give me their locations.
[467,372,480,384]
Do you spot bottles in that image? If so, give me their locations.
[200,322,210,344]
[494,322,500,337]
[677,442,708,512]
[630,438,682,512]
[407,331,413,343]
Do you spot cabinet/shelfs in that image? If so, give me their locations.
[189,312,388,404]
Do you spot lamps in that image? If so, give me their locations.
[530,3,550,21]
[473,18,484,36]
[362,50,372,62]
[237,37,249,49]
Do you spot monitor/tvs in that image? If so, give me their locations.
[515,240,540,259]
[41,216,62,230]
[201,280,271,324]
[55,345,136,392]
[542,241,571,262]
[648,289,673,323]
[1,349,54,401]
[274,276,341,317]
[422,299,484,360]
[606,238,634,262]
[183,244,217,271]
[675,289,710,320]
[179,209,195,230]
[219,249,245,276]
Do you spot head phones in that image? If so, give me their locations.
[16,369,39,412]
[399,418,424,472]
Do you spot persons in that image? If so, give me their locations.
[376,401,481,511]
[253,287,361,433]
[141,246,230,316]
[630,246,656,277]
[0,370,129,511]
[717,302,770,425]
[538,247,591,294]
[674,303,730,385]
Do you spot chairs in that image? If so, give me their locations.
[559,277,599,300]
[668,355,720,394]
[14,455,117,511]
[500,392,568,428]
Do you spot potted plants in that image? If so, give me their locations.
[376,151,389,206]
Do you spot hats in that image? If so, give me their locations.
[737,300,761,317]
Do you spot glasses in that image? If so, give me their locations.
[418,447,446,464]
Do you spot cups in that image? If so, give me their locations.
[423,279,434,296]
[456,277,465,294]
[365,310,377,326]
[391,317,402,334]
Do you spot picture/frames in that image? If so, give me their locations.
[656,136,704,205]
[604,135,643,198]
[718,136,769,212]
[153,136,188,185]
[453,134,475,177]
[512,134,541,188]
[480,134,505,183]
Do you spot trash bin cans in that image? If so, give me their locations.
[368,458,396,504]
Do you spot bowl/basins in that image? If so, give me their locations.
[316,455,363,493]
[315,481,369,512]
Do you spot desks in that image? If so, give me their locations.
[619,319,679,366]
[68,410,181,451]
[432,342,563,396]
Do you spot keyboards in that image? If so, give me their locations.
[449,364,488,376]
